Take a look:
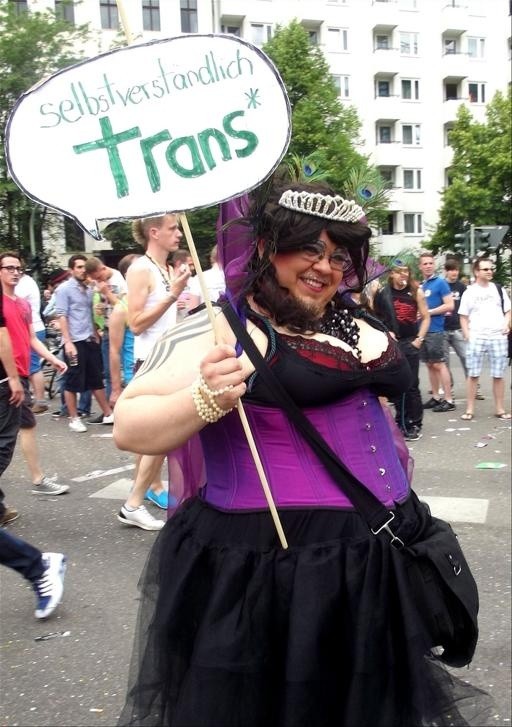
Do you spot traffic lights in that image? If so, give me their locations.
[455,229,469,251]
[473,229,491,254]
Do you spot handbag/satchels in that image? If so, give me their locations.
[395,503,480,671]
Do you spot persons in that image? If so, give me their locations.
[1,252,71,618]
[42,254,167,530]
[170,243,228,322]
[354,246,512,442]
[109,184,480,727]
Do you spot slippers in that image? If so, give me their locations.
[461,382,512,420]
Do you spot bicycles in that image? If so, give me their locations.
[41,333,105,398]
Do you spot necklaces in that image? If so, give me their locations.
[267,301,362,361]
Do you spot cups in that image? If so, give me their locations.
[69,357,81,368]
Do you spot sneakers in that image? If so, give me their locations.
[28,403,115,434]
[145,487,178,510]
[423,397,456,413]
[29,551,68,619]
[32,477,70,497]
[116,502,167,532]
[0,509,19,527]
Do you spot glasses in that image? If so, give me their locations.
[300,238,355,271]
[478,268,494,271]
[0,265,25,274]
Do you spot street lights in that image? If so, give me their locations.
[468,222,503,281]
